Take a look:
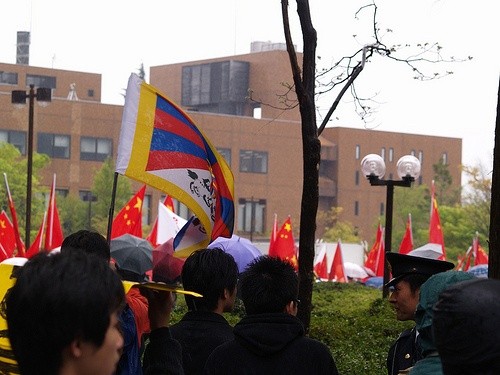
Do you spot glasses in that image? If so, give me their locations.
[294,298,301,306]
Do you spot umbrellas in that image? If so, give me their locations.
[406,242,443,260]
[109,234,154,274]
[466,265,489,277]
[0,255,29,268]
[206,232,264,275]
[153,237,193,279]
[342,263,369,280]
[365,277,383,287]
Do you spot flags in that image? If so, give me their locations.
[397,213,414,256]
[427,179,447,261]
[0,72,389,286]
[455,232,492,273]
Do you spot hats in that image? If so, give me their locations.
[383,252,455,287]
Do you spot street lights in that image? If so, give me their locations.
[82,193,98,233]
[12,83,53,253]
[360,153,421,300]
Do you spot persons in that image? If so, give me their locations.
[5,242,127,375]
[128,280,188,375]
[167,245,238,375]
[380,251,499,375]
[201,253,341,375]
[1,229,142,375]
